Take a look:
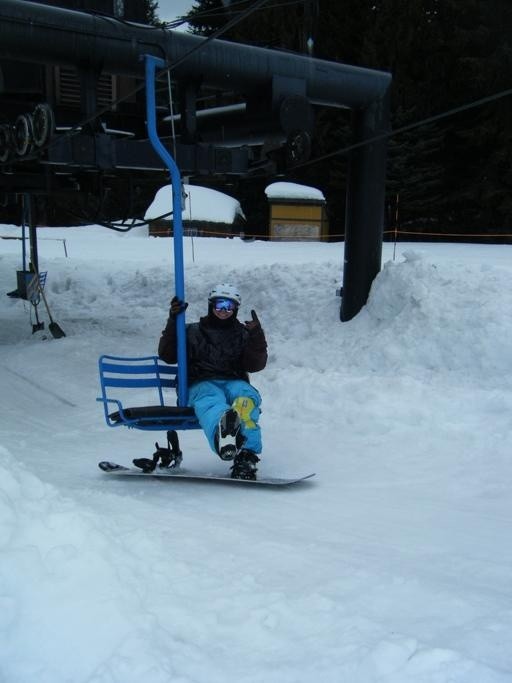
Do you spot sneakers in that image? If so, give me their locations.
[230,448,259,481]
[216,409,241,460]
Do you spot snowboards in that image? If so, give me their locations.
[100,429,316,485]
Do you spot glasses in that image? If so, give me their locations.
[211,298,237,314]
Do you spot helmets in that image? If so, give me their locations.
[208,283,241,306]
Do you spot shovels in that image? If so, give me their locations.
[30,258,66,339]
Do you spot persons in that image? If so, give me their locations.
[158,282,268,481]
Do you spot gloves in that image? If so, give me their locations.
[242,309,265,347]
[169,295,188,319]
